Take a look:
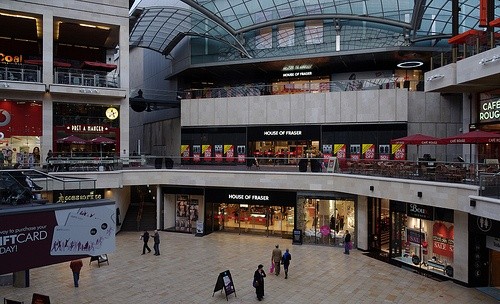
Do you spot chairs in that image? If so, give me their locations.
[345,159,466,184]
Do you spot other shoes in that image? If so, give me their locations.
[258,297,264,301]
[147,251,151,253]
[141,254,145,255]
[153,254,160,256]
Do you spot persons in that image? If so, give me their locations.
[46,149,139,171]
[281,249,291,279]
[229,207,344,234]
[344,230,352,254]
[70,260,83,287]
[140,229,151,256]
[271,245,282,276]
[345,74,362,91]
[253,265,266,302]
[149,230,160,256]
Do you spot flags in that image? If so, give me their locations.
[480,0,487,26]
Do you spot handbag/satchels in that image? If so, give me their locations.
[269,261,275,273]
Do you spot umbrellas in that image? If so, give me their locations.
[389,130,500,176]
[57,135,86,157]
[448,19,500,44]
[24,59,118,74]
[84,135,117,160]
